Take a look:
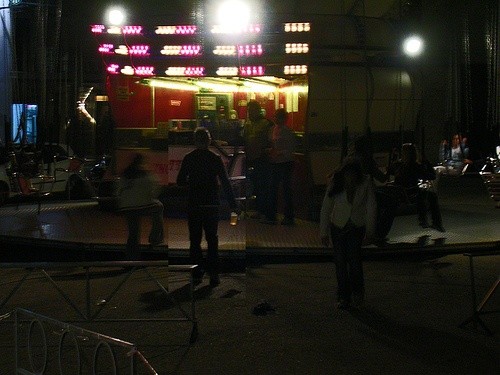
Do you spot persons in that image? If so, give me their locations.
[256,108,298,226]
[332,133,471,250]
[319,163,371,308]
[118,154,164,257]
[242,101,277,220]
[176,128,241,289]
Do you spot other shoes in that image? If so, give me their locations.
[373,237,391,246]
[282,217,295,225]
[192,273,223,286]
[338,293,366,306]
[259,217,278,224]
[419,223,447,233]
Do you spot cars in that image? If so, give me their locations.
[0,143,85,205]
